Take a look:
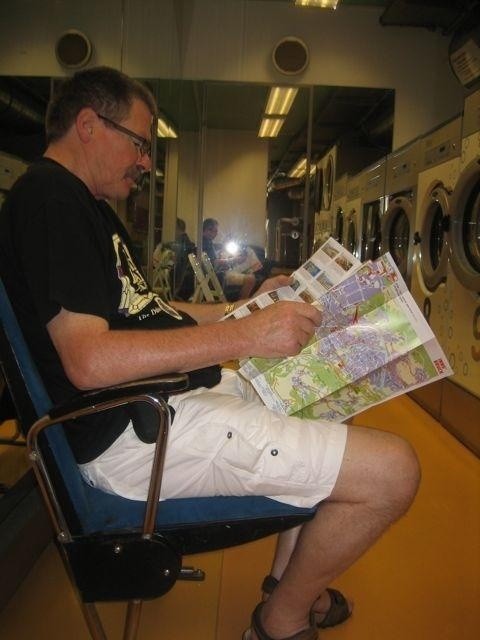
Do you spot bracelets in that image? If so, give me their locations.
[224,302,234,316]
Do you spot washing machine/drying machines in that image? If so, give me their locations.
[314,87,480,458]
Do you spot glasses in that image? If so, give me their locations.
[96,114,150,158]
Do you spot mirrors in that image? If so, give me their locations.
[0,73,395,303]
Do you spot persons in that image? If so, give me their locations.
[1,64,421,640]
[171,218,198,303]
[202,216,256,301]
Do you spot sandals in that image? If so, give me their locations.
[244,574,352,640]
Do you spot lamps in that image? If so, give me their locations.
[257,86,300,140]
[149,113,178,139]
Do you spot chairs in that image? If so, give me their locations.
[0,282,319,640]
